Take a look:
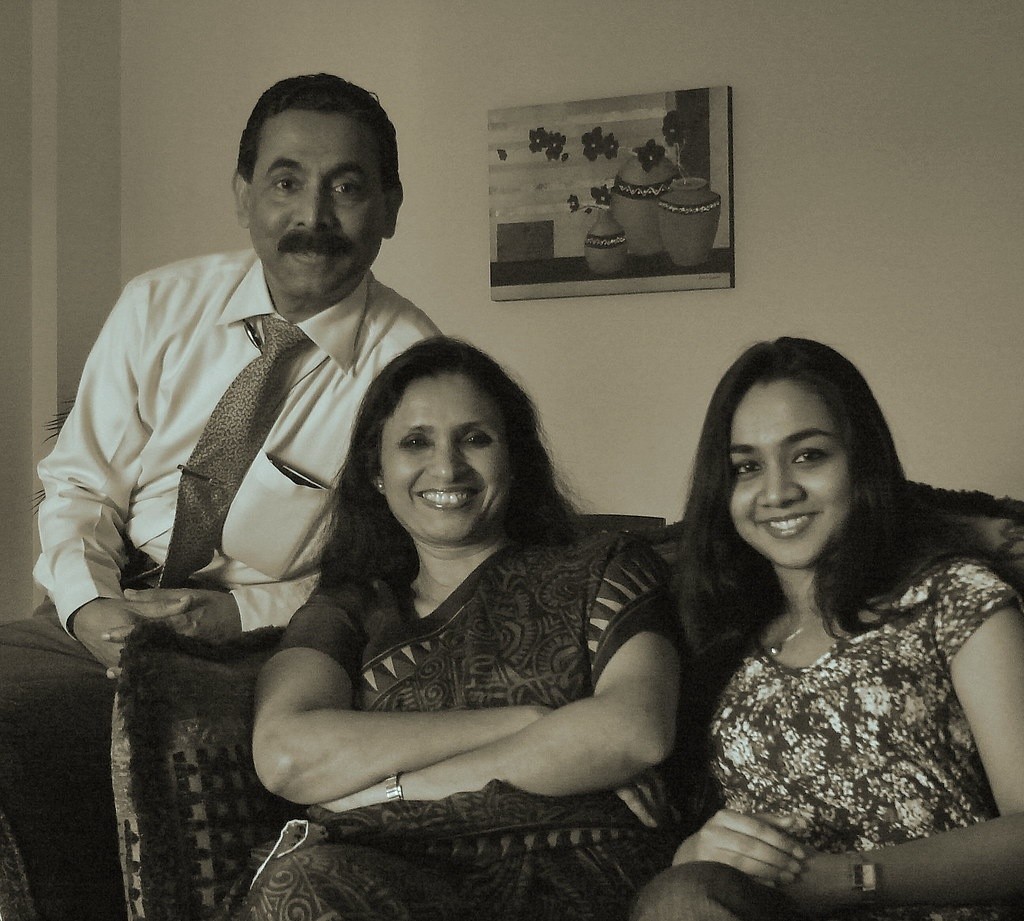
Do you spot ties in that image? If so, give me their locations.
[128,315,316,590]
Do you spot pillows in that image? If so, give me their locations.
[907,481,1024,594]
[108,621,282,921]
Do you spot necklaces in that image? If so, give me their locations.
[770,620,816,657]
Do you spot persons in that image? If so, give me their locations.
[215,339,682,921]
[0,72,447,921]
[631,338,1024,921]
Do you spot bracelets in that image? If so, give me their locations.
[849,851,878,895]
[386,775,405,802]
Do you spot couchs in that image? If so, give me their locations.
[0,514,1024,921]
